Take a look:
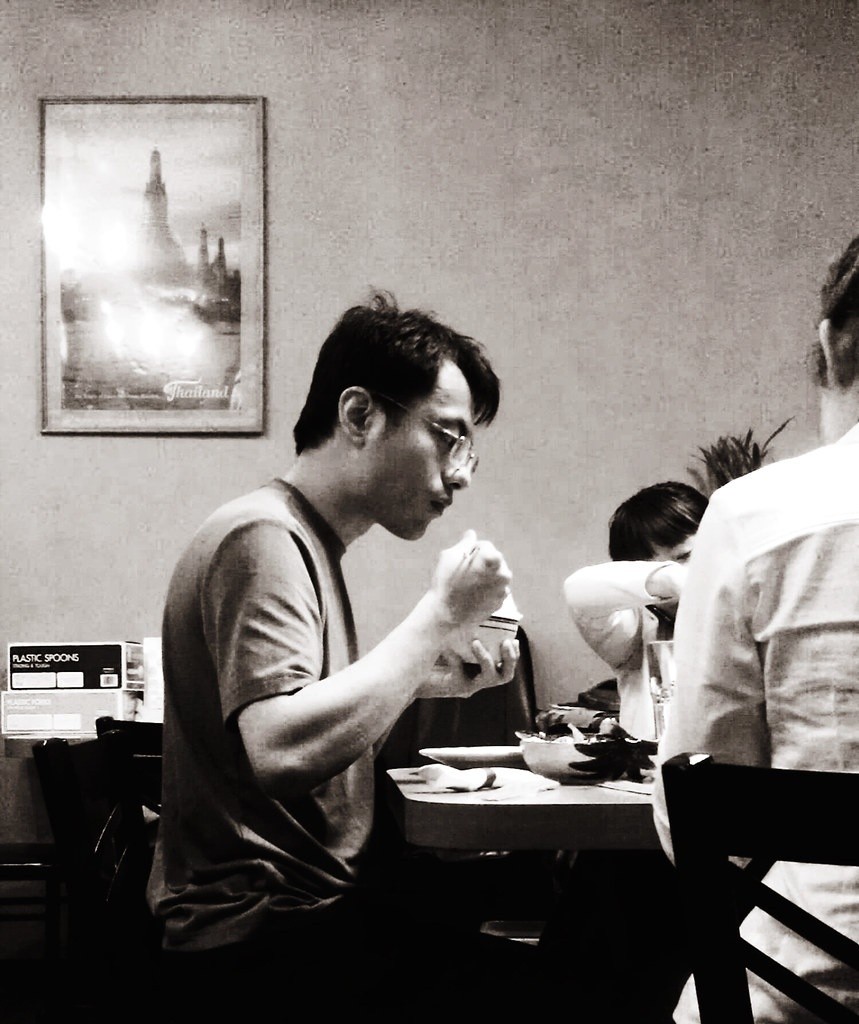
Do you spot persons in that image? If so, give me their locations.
[651,231,859,1024]
[562,480,710,743]
[147,303,521,950]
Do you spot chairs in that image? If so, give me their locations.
[34,719,165,1012]
[664,756,859,1023]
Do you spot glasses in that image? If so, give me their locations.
[379,392,479,475]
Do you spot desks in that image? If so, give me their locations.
[382,756,657,1024]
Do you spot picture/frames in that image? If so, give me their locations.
[39,91,272,440]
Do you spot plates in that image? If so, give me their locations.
[419,746,528,771]
[520,734,630,786]
